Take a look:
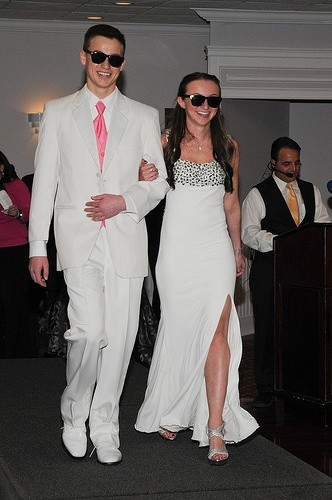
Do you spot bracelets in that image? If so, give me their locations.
[14,209,22,219]
[234,249,241,255]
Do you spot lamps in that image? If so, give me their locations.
[27,112,43,134]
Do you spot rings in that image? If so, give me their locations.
[150,168,154,172]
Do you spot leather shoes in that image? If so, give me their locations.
[60,421,88,460]
[90,443,124,466]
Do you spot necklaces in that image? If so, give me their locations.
[191,137,211,150]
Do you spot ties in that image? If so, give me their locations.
[287,184,300,226]
[92,101,108,170]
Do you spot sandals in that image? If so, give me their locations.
[206,420,230,465]
[157,428,178,441]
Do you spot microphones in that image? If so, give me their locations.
[274,168,294,178]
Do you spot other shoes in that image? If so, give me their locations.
[252,392,277,408]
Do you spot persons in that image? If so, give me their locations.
[0,151,32,319]
[241,137,329,407]
[29,24,169,463]
[135,72,260,461]
[31,65,161,364]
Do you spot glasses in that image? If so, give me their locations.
[85,49,124,67]
[184,93,222,108]
